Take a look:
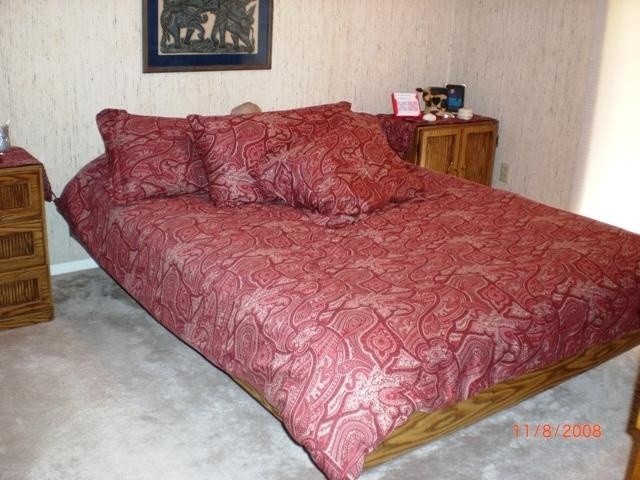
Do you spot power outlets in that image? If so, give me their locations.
[501,164,510,183]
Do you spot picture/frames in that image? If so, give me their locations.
[141,0,274,74]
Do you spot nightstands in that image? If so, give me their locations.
[378,110,500,187]
[0,146,54,331]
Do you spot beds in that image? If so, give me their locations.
[58,101,639,476]
